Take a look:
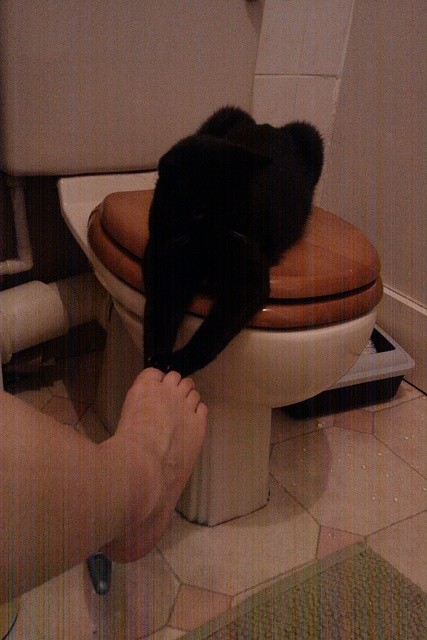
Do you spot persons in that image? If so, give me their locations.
[0,368,209,606]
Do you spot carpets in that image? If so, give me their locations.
[175,539,426,639]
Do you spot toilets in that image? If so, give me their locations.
[56,174,383,525]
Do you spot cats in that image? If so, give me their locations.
[139,101,326,382]
